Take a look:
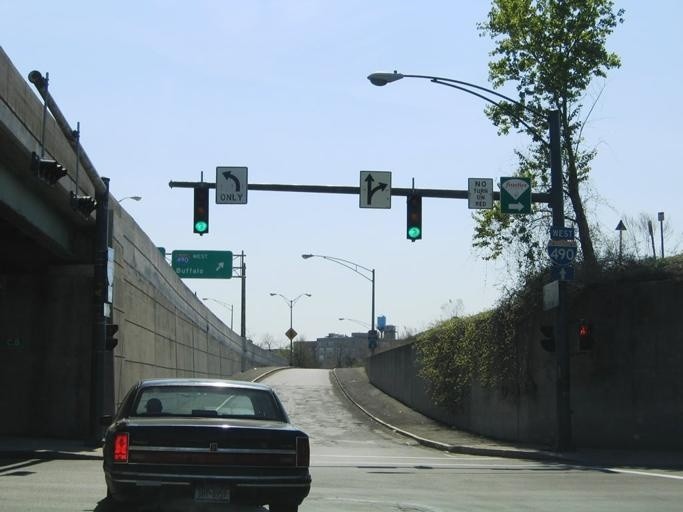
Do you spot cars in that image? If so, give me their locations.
[102,376,312,511]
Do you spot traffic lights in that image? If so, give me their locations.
[193,182,209,236]
[539,324,555,355]
[32,155,67,187]
[575,319,596,356]
[72,192,100,220]
[406,191,422,242]
[100,321,119,351]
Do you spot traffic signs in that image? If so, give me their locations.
[215,166,248,204]
[546,227,577,283]
[469,176,531,214]
[171,250,234,280]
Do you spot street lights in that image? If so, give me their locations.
[367,69,572,449]
